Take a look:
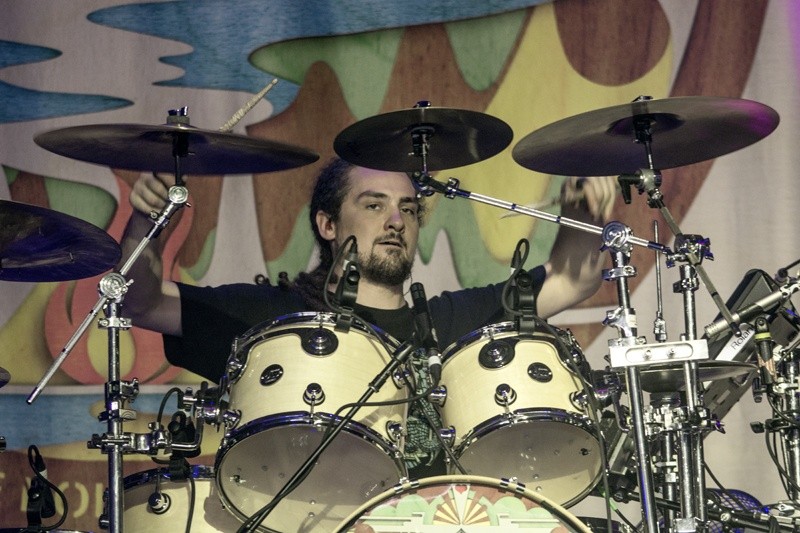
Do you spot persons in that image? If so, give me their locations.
[113,158,620,395]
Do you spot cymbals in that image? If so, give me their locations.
[512,94,782,179]
[332,107,516,174]
[0,197,124,283]
[613,359,763,390]
[33,121,321,177]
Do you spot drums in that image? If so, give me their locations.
[213,311,418,532]
[436,318,612,513]
[325,472,592,533]
[106,465,261,532]
